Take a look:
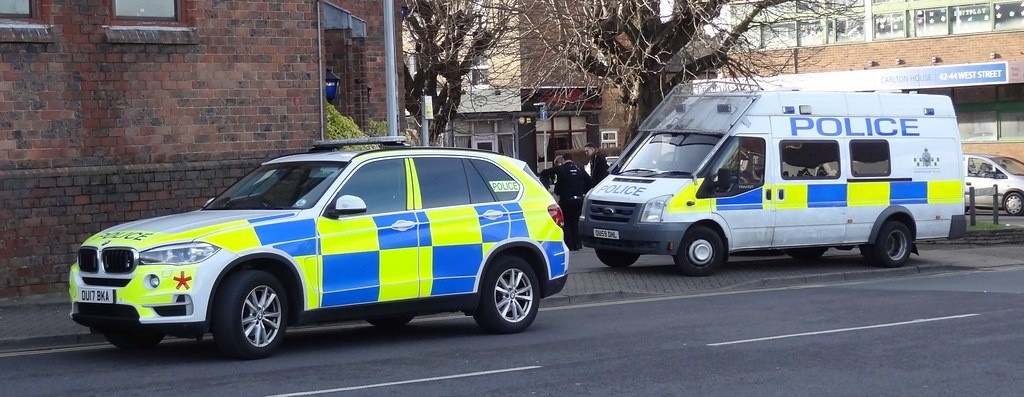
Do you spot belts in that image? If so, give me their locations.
[571,196,582,199]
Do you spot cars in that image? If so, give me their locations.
[584,156,618,176]
[963,151,1024,215]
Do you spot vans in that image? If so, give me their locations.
[576,79,970,276]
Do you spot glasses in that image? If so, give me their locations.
[585,149,589,152]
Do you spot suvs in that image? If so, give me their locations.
[66,134,571,361]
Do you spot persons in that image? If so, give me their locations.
[553,154,593,252]
[585,143,610,186]
[538,155,564,206]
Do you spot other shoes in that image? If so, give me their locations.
[578,242,582,249]
[568,244,579,251]
